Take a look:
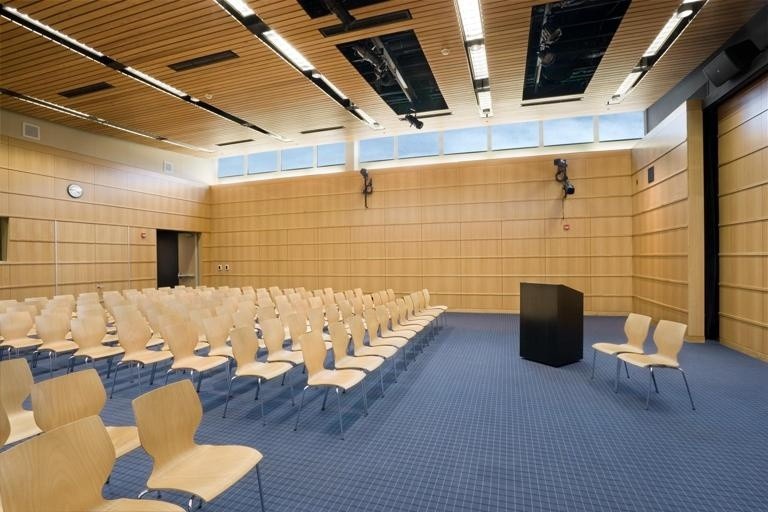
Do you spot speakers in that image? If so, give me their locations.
[704,39,761,87]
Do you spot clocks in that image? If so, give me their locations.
[67,183,84,198]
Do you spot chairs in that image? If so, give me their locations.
[590,312,696,411]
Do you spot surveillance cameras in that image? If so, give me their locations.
[564,184,574,194]
[361,169,368,176]
[554,159,567,167]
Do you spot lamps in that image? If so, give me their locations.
[405,107,423,129]
[360,167,374,209]
[553,156,576,201]
